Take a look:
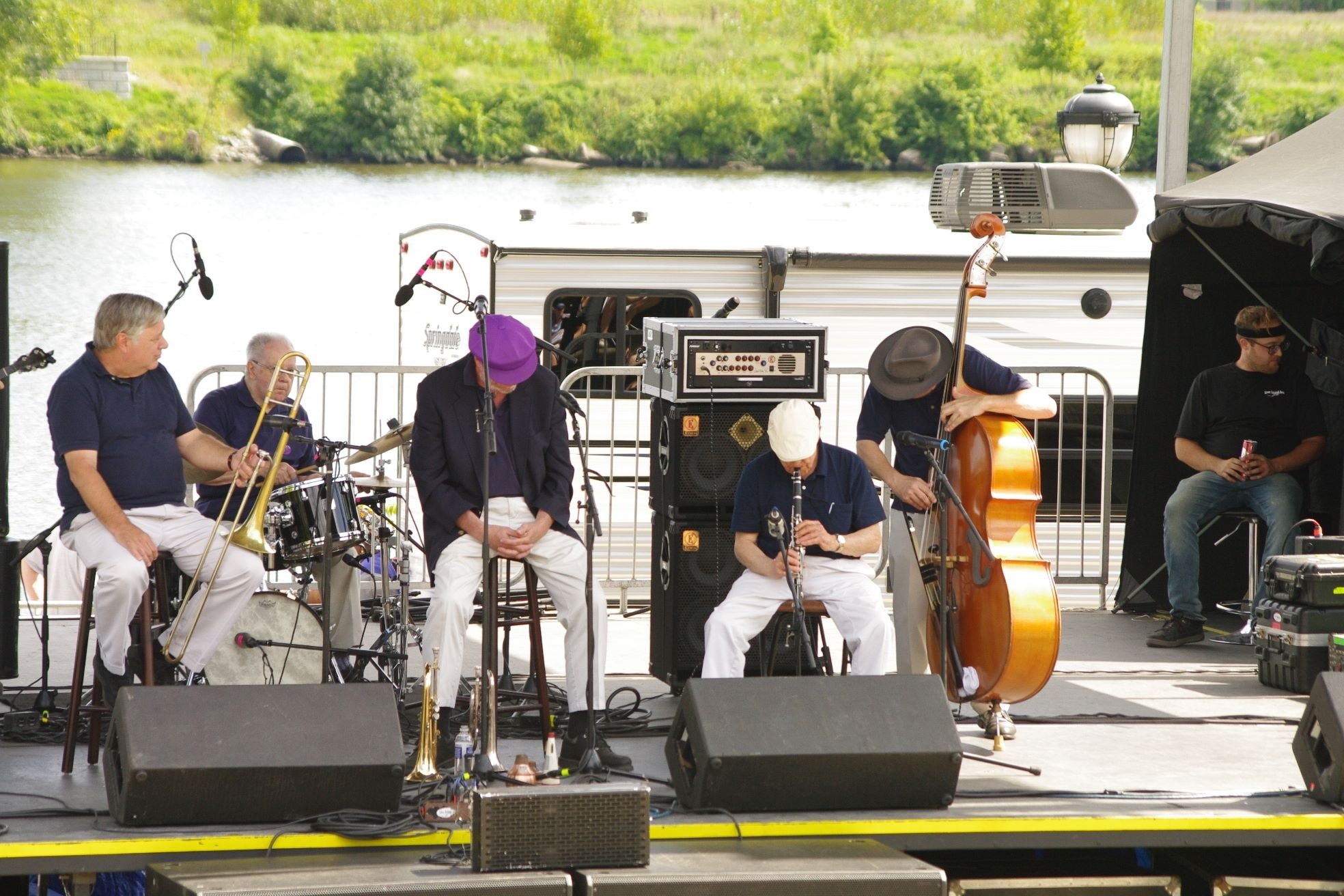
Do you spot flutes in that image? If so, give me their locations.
[787,465,805,646]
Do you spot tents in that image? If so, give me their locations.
[1111,105,1344,613]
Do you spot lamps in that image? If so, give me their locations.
[1056,74,1140,176]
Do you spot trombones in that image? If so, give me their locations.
[162,349,313,667]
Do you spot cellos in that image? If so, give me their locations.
[926,213,1063,753]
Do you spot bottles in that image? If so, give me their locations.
[454,725,474,777]
[506,755,535,786]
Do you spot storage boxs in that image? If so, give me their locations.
[1264,553,1344,609]
[1254,598,1344,695]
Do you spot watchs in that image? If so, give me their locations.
[832,533,845,553]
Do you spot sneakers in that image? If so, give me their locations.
[1145,615,1205,646]
[978,707,1017,740]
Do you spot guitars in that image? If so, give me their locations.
[0,347,48,381]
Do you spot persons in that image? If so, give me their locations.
[682,400,895,762]
[1146,305,1325,650]
[856,327,1058,740]
[194,333,369,683]
[46,293,266,707]
[404,315,635,779]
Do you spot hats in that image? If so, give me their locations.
[468,314,538,384]
[867,325,955,400]
[768,399,820,463]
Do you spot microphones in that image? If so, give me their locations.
[235,633,259,648]
[192,238,213,300]
[768,506,785,537]
[395,251,437,306]
[342,553,372,574]
[474,294,488,320]
[355,492,395,506]
[896,431,953,451]
[314,443,325,467]
[554,390,586,417]
[713,296,741,318]
[262,415,312,428]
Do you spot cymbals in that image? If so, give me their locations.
[344,420,415,466]
[351,476,417,489]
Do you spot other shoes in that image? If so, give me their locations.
[92,654,130,712]
[126,645,175,685]
[328,652,364,682]
[405,733,453,775]
[558,728,632,773]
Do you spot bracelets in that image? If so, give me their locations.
[228,449,237,471]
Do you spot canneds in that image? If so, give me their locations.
[1239,439,1258,479]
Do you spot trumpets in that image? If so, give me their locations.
[468,664,508,773]
[404,646,443,783]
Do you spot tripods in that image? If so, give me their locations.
[343,275,672,793]
[0,521,67,725]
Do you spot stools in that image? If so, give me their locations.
[456,555,550,759]
[1208,511,1265,647]
[60,551,175,774]
[768,600,835,676]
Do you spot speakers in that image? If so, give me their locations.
[1294,534,1344,555]
[1291,671,1344,804]
[665,673,962,811]
[101,686,405,826]
[576,837,948,896]
[0,535,19,680]
[647,398,820,521]
[649,512,818,689]
[471,781,650,871]
[0,241,10,538]
[144,850,574,896]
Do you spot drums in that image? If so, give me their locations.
[267,472,369,562]
[153,551,203,620]
[201,590,331,687]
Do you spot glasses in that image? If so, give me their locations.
[253,360,300,378]
[1246,338,1291,355]
